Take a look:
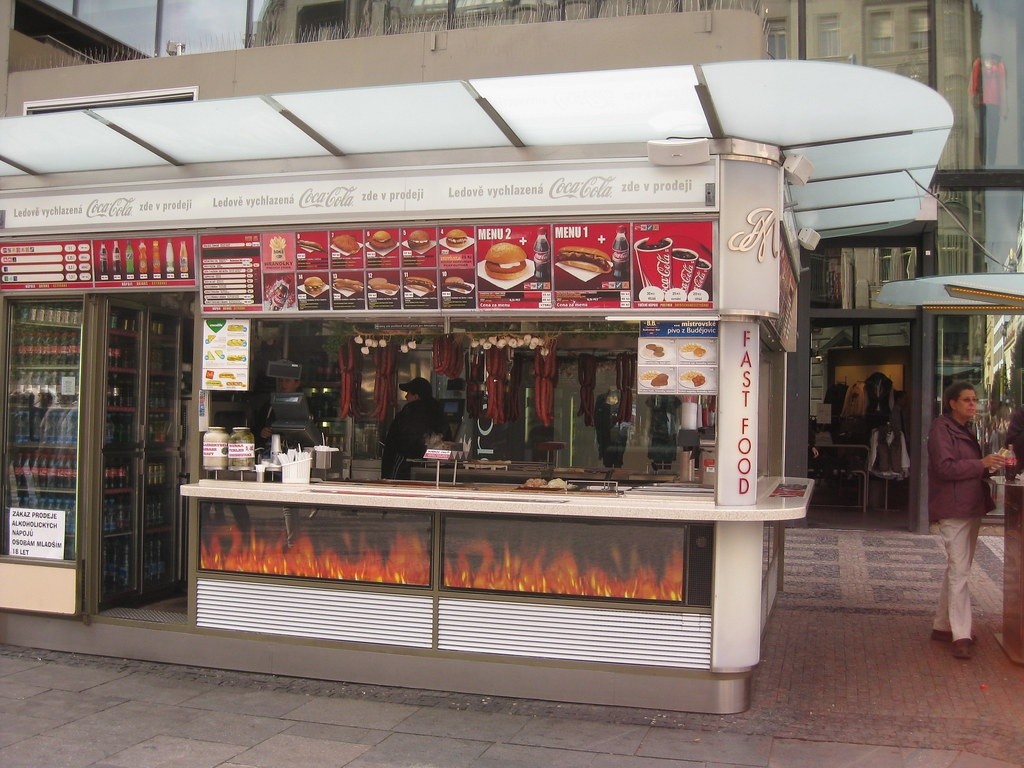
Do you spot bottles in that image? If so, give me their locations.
[100,238,190,273]
[10,327,79,533]
[203,426,229,467]
[533,227,549,282]
[1005,444,1017,481]
[106,335,172,590]
[228,427,256,467]
[611,225,629,283]
[269,274,292,311]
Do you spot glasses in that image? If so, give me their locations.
[954,396,981,405]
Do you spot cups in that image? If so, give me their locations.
[634,237,711,294]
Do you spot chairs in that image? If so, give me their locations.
[538,440,566,467]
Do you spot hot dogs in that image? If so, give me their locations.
[298,239,326,259]
[335,275,474,301]
[987,447,1010,477]
[557,245,614,274]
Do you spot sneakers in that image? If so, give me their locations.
[952,638,973,661]
[931,626,957,642]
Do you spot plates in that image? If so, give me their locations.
[678,371,708,389]
[297,283,329,297]
[301,246,314,253]
[368,284,400,296]
[402,239,437,255]
[639,371,669,389]
[366,241,399,257]
[404,284,436,297]
[446,282,475,294]
[439,236,474,253]
[639,344,669,360]
[555,259,614,282]
[333,285,363,297]
[330,240,363,256]
[477,258,536,290]
[678,343,707,359]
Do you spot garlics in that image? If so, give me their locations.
[468,333,549,356]
[351,332,417,353]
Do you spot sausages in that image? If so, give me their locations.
[333,346,395,421]
[431,332,463,378]
[482,348,632,427]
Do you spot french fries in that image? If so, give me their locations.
[269,236,287,262]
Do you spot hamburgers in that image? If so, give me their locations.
[484,242,526,279]
[302,277,325,294]
[369,227,469,260]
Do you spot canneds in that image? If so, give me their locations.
[14,240,190,584]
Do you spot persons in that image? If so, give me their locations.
[380,376,455,480]
[978,51,1008,156]
[593,385,632,467]
[807,440,821,460]
[927,379,1007,659]
[251,377,317,462]
[1004,406,1024,475]
[646,394,683,469]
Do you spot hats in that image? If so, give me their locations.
[397,377,433,396]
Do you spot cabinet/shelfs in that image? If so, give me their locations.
[809,444,870,515]
[6,297,187,611]
